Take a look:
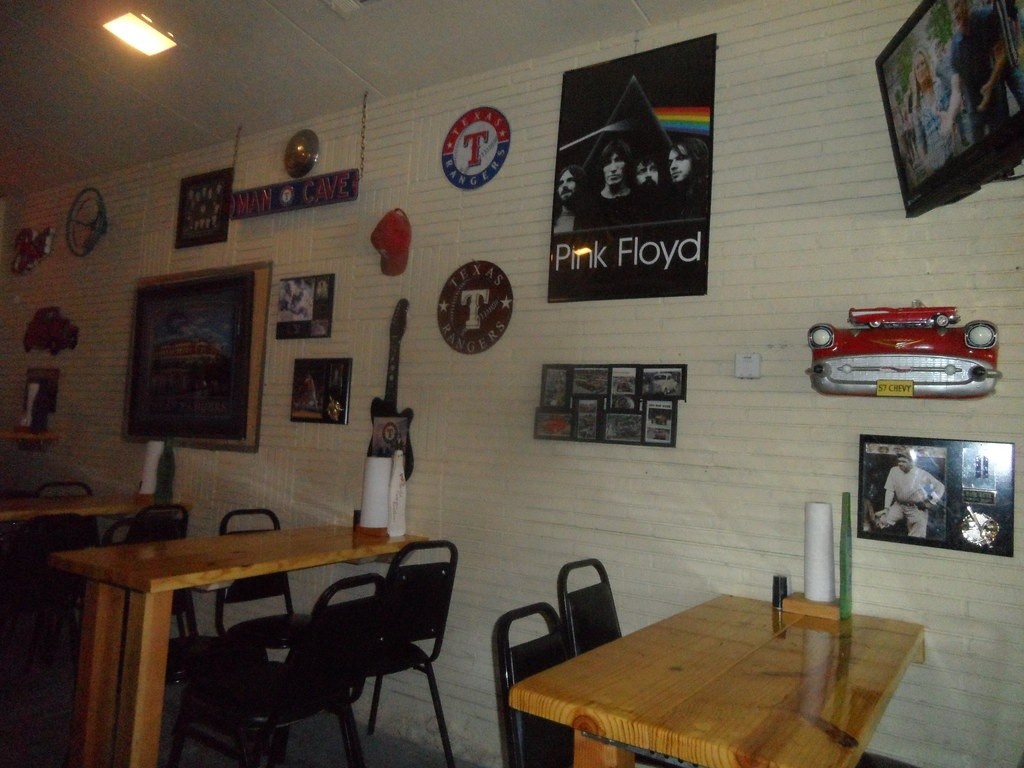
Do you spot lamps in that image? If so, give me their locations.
[573,241,593,257]
[102,9,178,57]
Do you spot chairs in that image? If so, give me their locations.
[558,557,678,768]
[0,481,459,768]
[491,602,574,768]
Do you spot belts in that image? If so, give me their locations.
[897,500,916,506]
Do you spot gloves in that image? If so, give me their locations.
[916,502,928,512]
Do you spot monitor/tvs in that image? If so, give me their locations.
[875,0,1024,218]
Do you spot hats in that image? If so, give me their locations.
[897,451,911,460]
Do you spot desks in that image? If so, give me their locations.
[46,528,429,767]
[0,494,192,565]
[510,593,924,768]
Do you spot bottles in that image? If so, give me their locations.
[387,450,407,538]
[353,510,361,531]
[772,576,787,609]
[839,493,852,618]
[154,436,175,504]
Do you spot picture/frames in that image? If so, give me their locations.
[172,169,235,249]
[532,364,687,447]
[122,260,273,453]
[858,434,1015,559]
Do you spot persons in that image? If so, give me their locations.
[864,444,946,537]
[888,0,1024,186]
[296,365,343,412]
[555,137,708,233]
